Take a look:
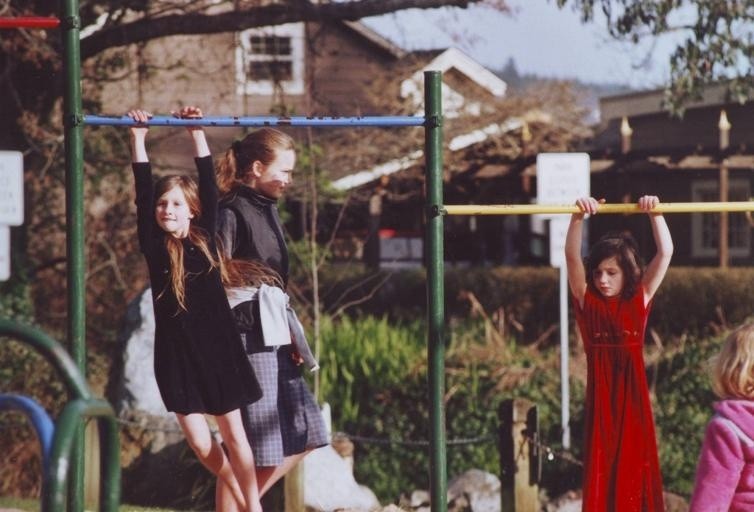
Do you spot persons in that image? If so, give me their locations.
[564,194,676,511]
[688,315,754,511]
[211,125,332,512]
[127,106,266,512]
